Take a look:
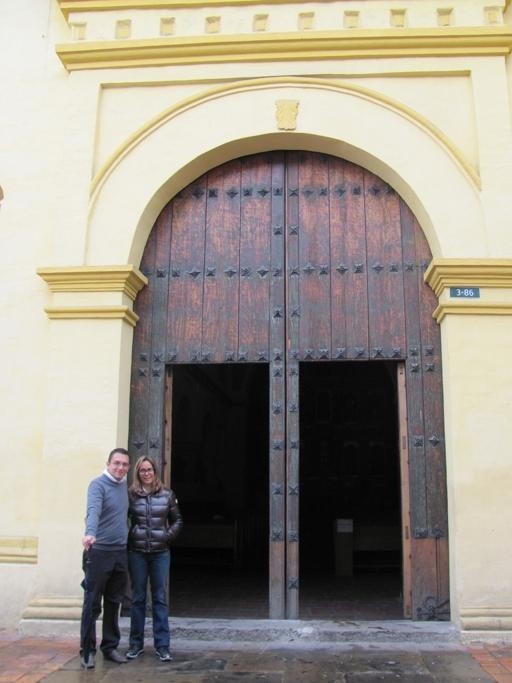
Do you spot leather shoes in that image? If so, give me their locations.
[104,648,129,662]
[80,653,95,668]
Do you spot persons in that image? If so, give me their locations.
[77,448,131,668]
[127,456,182,661]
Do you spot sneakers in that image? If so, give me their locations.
[156,646,173,661]
[126,647,144,659]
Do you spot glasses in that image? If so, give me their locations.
[137,467,152,474]
